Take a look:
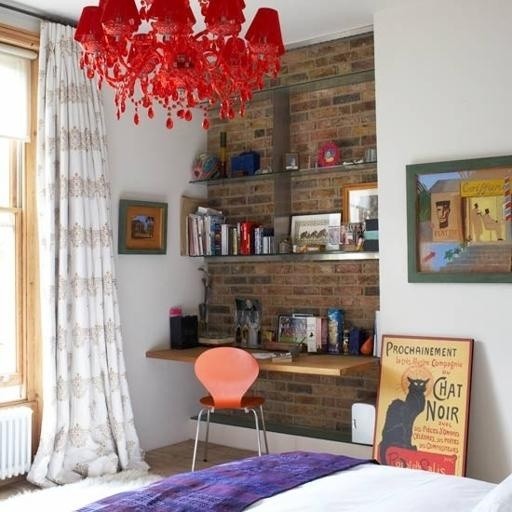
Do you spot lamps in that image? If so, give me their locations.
[74,0,284,133]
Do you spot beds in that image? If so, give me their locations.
[69,450,512,511]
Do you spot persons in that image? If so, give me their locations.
[483,208,504,241]
[472,203,484,242]
[436,200,451,229]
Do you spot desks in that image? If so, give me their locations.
[146,340,379,378]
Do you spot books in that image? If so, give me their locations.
[179,194,275,257]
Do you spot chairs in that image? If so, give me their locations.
[189,346,270,469]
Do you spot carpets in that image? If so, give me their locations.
[0,465,166,512]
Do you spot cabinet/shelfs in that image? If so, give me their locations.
[183,66,381,265]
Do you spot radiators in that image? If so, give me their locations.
[1,404,37,481]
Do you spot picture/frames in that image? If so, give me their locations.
[341,180,379,252]
[287,211,344,252]
[404,152,512,284]
[371,333,475,477]
[319,140,340,168]
[275,314,318,344]
[282,151,300,172]
[116,197,171,257]
